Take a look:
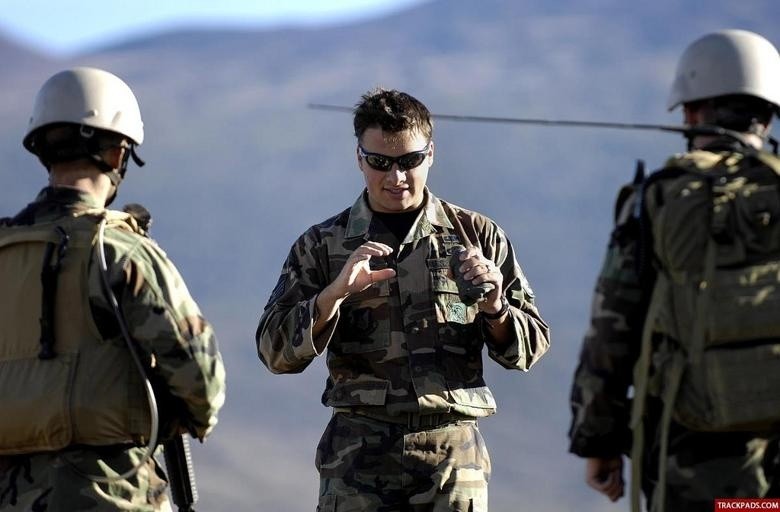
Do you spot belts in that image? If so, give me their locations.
[333,406,476,431]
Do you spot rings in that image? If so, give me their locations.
[486,264,492,273]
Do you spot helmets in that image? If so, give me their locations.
[23,67,144,154]
[668,29,780,110]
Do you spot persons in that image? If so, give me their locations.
[255,85,551,512]
[565,26,779,510]
[0,64,228,511]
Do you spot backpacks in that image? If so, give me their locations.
[646,146,780,433]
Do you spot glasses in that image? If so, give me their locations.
[358,144,429,171]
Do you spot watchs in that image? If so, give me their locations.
[479,294,510,320]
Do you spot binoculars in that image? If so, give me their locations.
[449,244,495,307]
